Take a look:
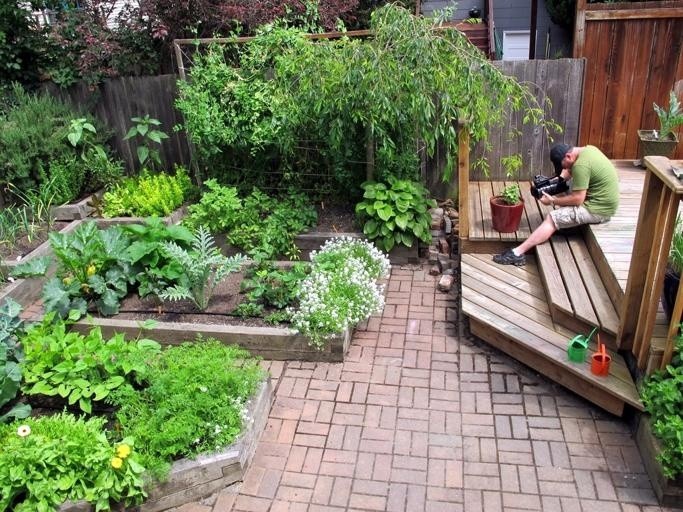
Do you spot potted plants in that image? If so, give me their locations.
[661,211,683,336]
[637,89,683,168]
[490,183,525,233]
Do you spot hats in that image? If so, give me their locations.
[548,144,571,176]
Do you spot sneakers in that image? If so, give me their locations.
[492,249,526,267]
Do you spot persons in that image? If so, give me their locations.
[492,143,621,266]
